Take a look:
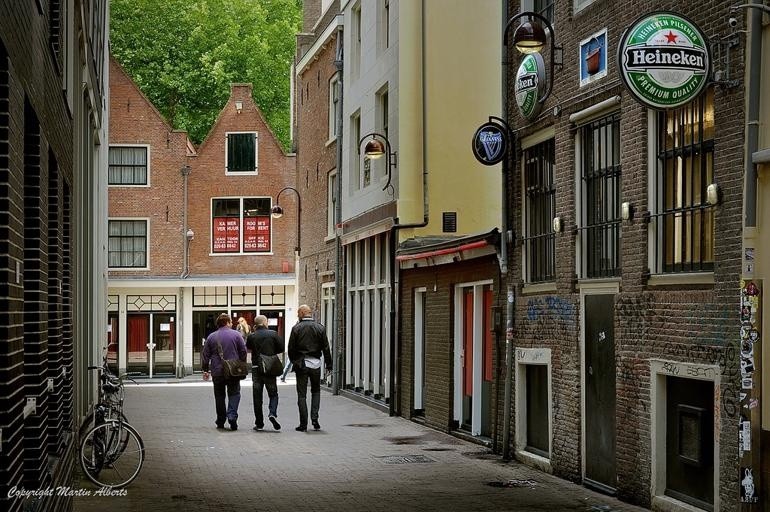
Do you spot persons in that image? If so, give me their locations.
[237,317,251,345]
[202,314,247,430]
[246,315,284,431]
[288,304,331,432]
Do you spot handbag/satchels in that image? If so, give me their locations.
[259,354,284,378]
[223,360,248,380]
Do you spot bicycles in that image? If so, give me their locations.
[78,342,146,489]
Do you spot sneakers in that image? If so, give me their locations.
[313,420,320,429]
[269,414,282,430]
[229,416,237,430]
[254,425,264,431]
[296,426,307,431]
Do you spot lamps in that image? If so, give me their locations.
[358,133,395,191]
[272,187,300,256]
[504,11,563,104]
